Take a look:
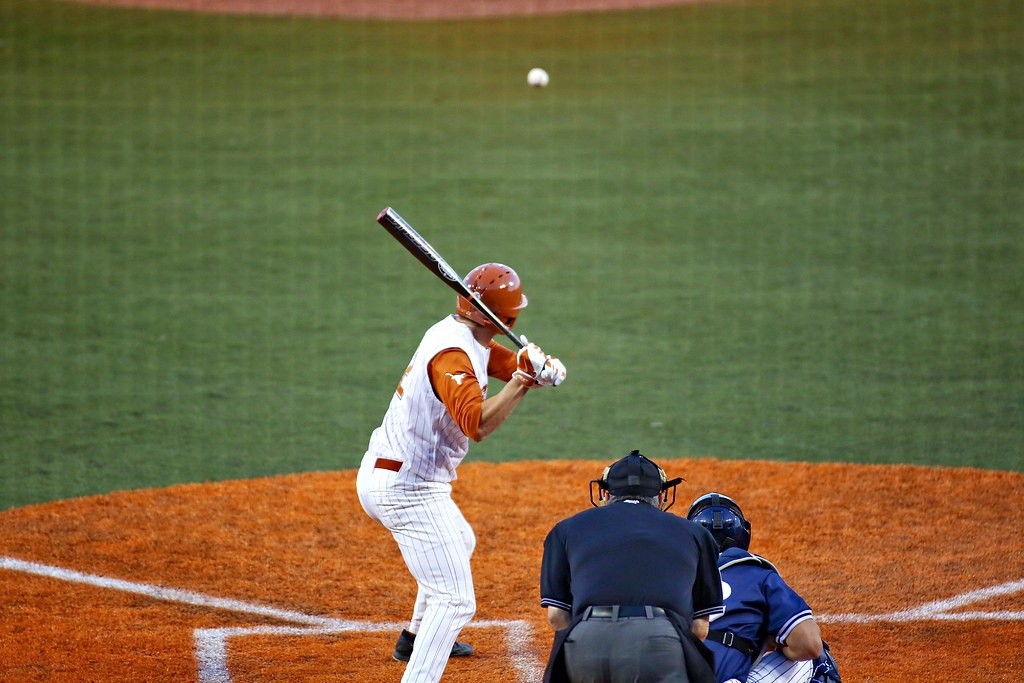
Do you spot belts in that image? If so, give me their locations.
[589,606,667,617]
[374,458,403,472]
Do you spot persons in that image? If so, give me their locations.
[540,450,822,683]
[354,263,567,683]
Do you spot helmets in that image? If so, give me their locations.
[687,493,751,552]
[456,263,528,335]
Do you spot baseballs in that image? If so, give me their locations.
[526,67,550,88]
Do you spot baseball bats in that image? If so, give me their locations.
[376,205,552,383]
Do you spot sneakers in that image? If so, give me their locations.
[393,629,471,661]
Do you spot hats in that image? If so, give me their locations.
[600,450,667,496]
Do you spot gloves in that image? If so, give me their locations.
[512,343,549,387]
[534,357,566,387]
